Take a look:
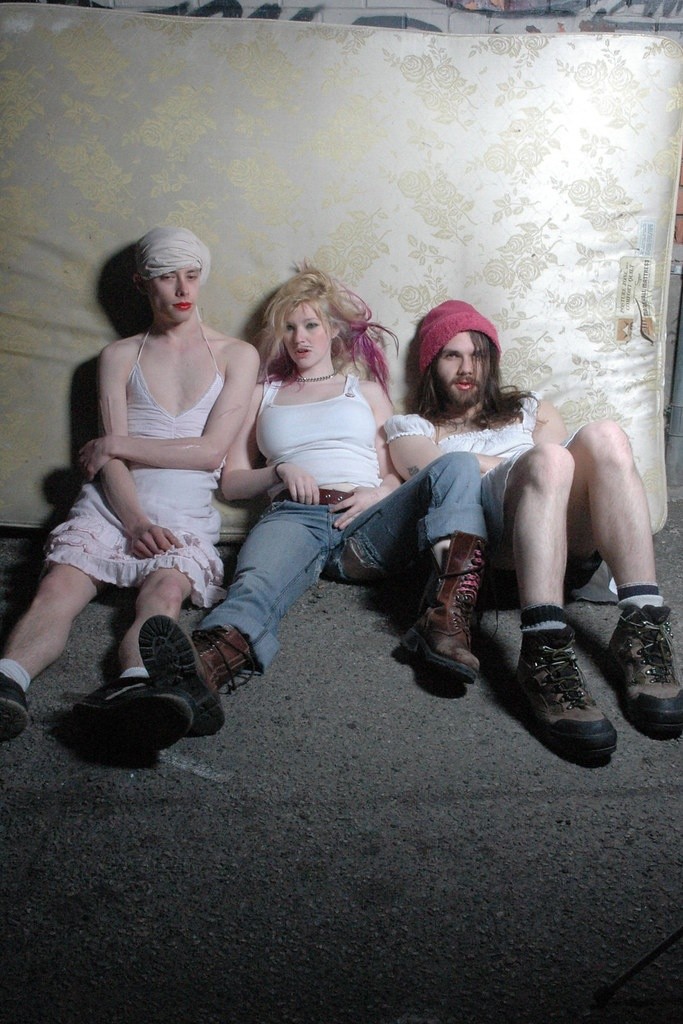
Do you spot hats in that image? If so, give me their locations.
[417,300,501,377]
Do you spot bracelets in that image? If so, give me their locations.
[274,461,287,483]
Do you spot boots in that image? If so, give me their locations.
[138,615,255,736]
[400,531,493,685]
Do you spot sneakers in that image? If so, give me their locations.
[601,605,683,739]
[74,677,194,759]
[512,630,617,758]
[0,672,28,740]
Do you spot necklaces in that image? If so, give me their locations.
[294,369,338,383]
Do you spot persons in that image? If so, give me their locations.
[0,224,261,752]
[385,299,683,768]
[136,262,490,736]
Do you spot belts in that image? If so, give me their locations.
[271,488,353,510]
